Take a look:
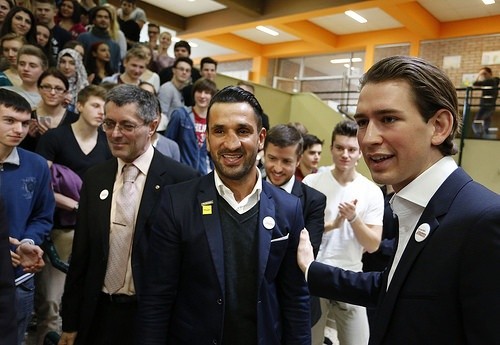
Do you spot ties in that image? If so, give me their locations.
[104,166,141,295]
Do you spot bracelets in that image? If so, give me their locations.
[348,214,357,223]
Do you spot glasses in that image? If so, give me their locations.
[39,83,66,94]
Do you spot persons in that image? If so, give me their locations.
[297,56,500,345]
[0,0,326,345]
[473,67,500,138]
